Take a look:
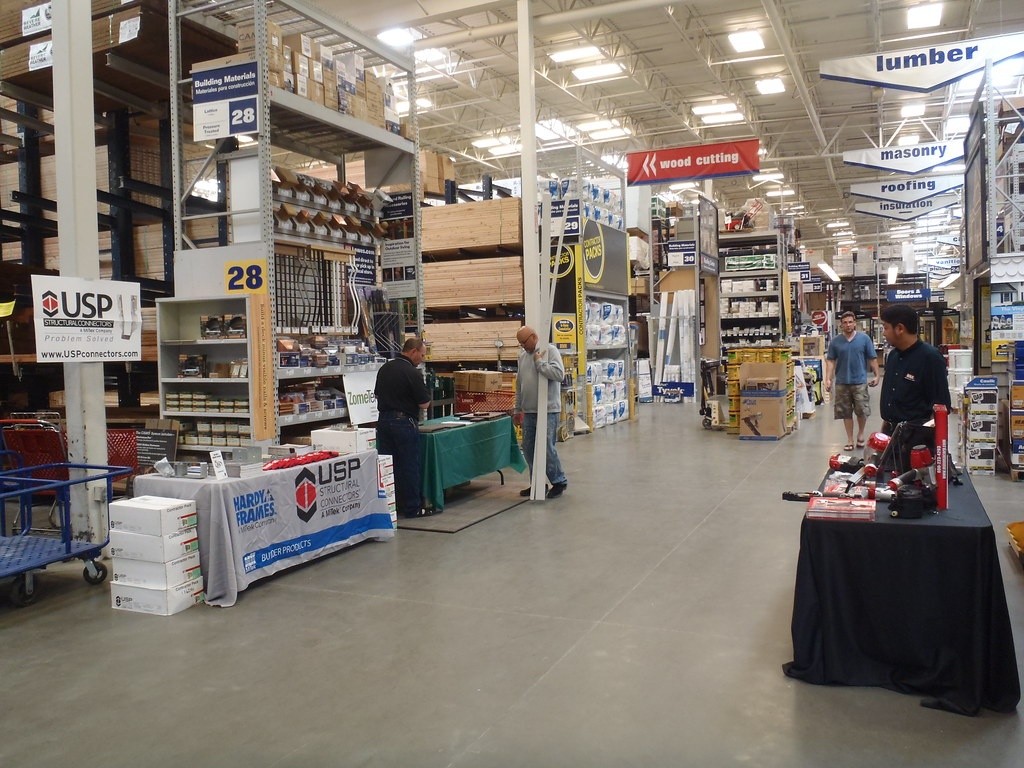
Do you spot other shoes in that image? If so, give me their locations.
[856,436,864,448]
[844,445,854,451]
[405,508,434,518]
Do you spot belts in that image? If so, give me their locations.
[380,411,408,416]
[886,422,898,432]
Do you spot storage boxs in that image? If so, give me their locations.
[109,528,198,563]
[666,202,683,217]
[652,197,666,219]
[376,455,397,532]
[1006,339,1024,471]
[800,336,823,357]
[238,21,416,142]
[958,378,998,476]
[110,576,205,616]
[109,494,197,536]
[739,389,787,440]
[311,427,376,454]
[111,552,200,590]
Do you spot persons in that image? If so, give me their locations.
[880,304,951,438]
[513,326,567,498]
[826,311,880,450]
[373,338,437,517]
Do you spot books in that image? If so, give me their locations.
[208,460,264,479]
[806,497,877,521]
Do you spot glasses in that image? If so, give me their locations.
[519,334,534,346]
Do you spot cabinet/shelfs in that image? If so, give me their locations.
[628,195,720,403]
[719,230,804,385]
[156,85,429,457]
[420,143,630,441]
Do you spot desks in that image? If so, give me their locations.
[801,467,993,663]
[133,448,379,588]
[375,412,511,511]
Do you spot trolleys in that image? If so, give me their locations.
[0,461,134,607]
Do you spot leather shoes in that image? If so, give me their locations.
[547,483,567,498]
[520,484,548,496]
